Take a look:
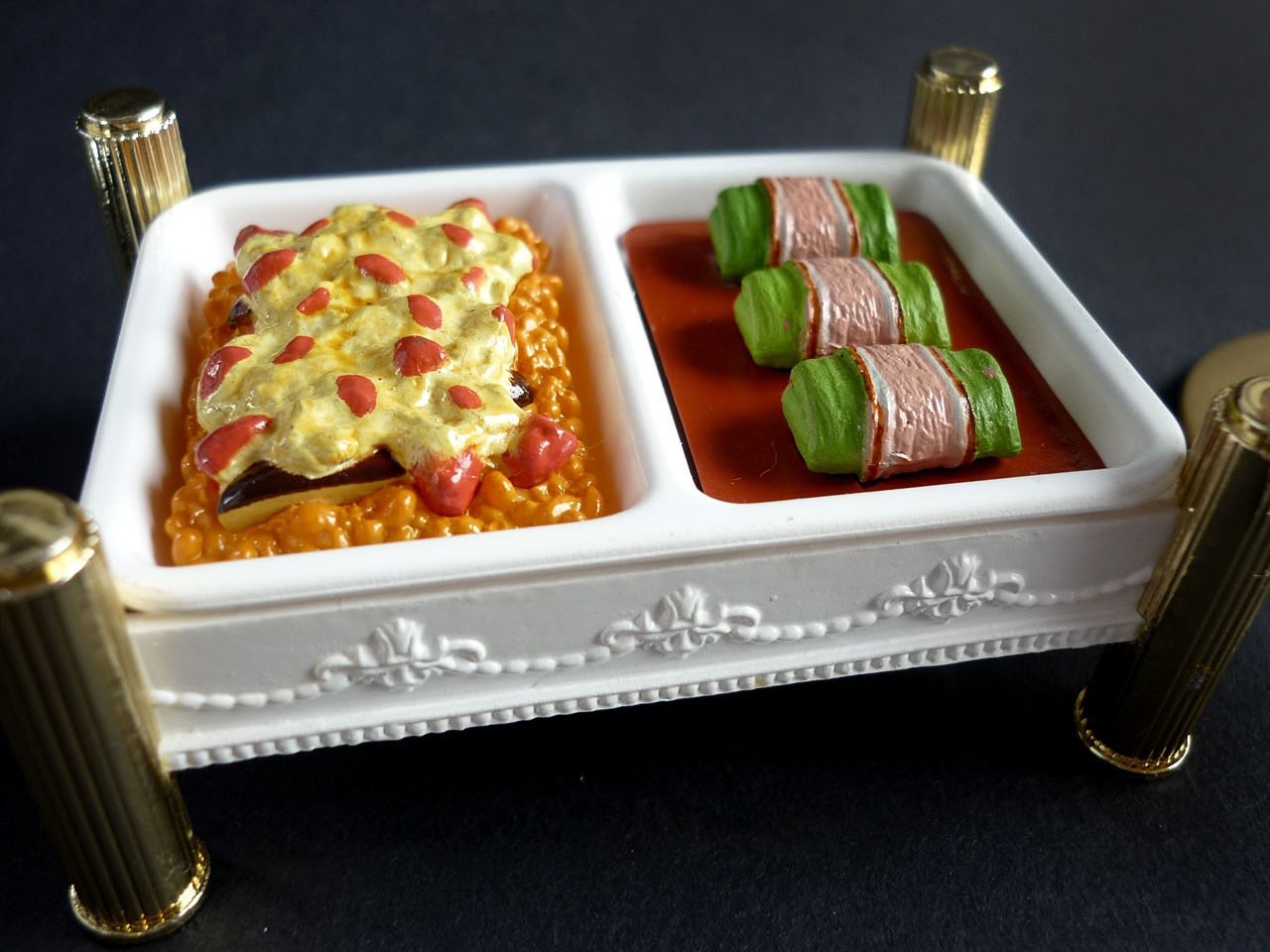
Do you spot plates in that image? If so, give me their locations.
[70,149,1188,619]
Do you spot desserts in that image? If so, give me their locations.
[153,117,1021,584]
